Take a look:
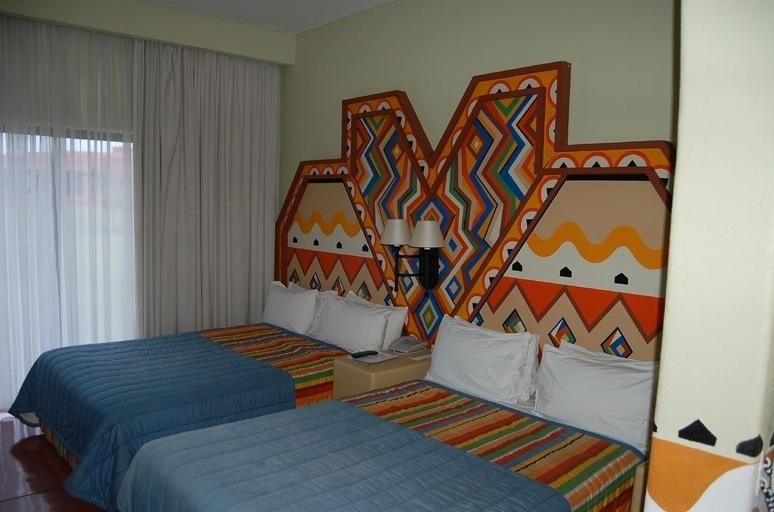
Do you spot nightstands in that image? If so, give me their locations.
[332,349,431,400]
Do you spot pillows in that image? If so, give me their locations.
[261,280,410,357]
[423,312,660,459]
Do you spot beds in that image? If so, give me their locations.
[118,380,648,512]
[37,323,352,512]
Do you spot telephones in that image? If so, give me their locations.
[388,336,425,353]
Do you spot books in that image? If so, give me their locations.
[346,352,399,365]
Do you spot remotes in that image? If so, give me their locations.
[351,350,378,358]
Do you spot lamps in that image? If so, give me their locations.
[379,219,446,297]
